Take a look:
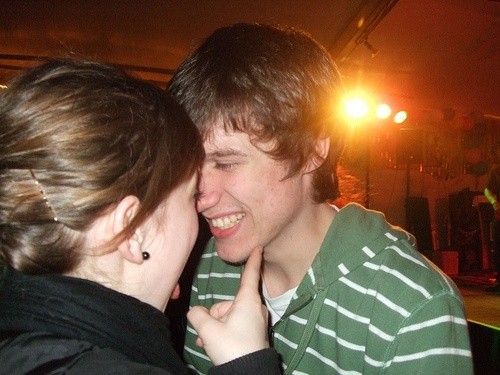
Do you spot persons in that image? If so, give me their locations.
[0,56,283,375]
[166,23,474,375]
